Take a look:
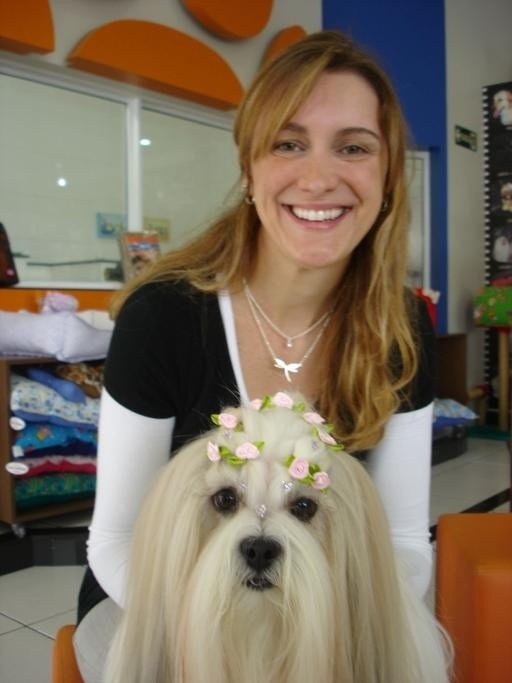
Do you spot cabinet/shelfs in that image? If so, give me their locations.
[430,332,468,466]
[1,353,106,538]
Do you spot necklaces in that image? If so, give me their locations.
[241,278,338,384]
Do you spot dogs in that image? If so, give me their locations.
[104,390,454,682]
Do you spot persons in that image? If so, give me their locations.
[79,32,438,608]
